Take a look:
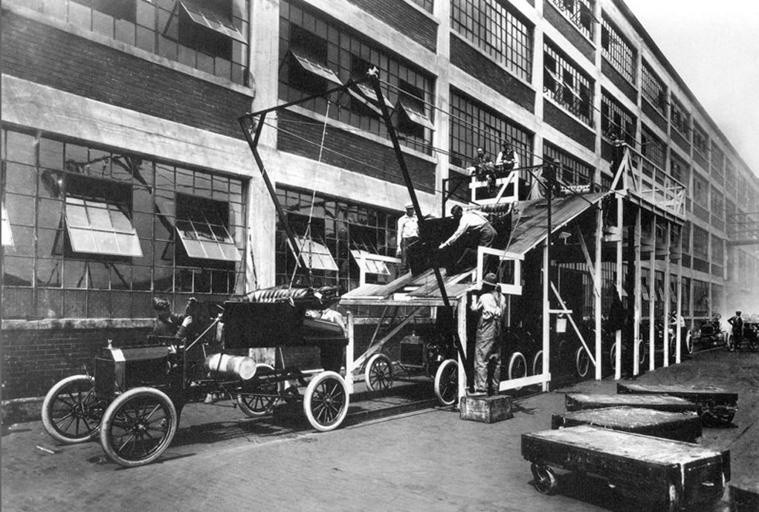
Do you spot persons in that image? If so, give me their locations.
[395,202,428,280]
[472,147,492,166]
[437,204,499,272]
[466,273,506,396]
[495,139,520,197]
[727,310,743,351]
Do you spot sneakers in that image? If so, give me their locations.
[466,388,500,397]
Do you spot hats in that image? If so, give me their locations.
[479,272,498,288]
[500,139,511,146]
[404,201,415,210]
